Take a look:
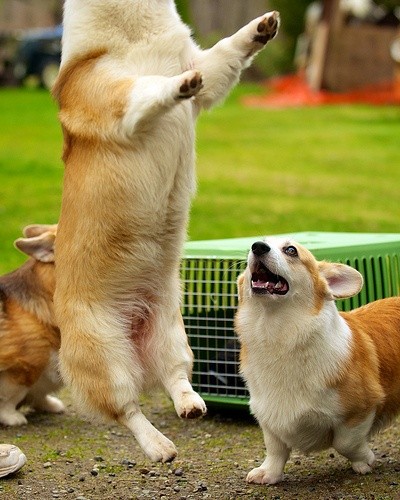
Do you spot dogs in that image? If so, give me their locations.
[53,0,282,463]
[230,238,400,484]
[0,223,67,427]
